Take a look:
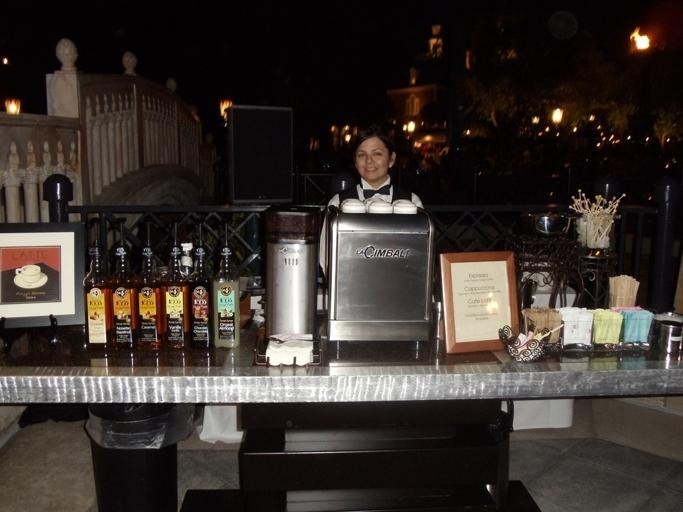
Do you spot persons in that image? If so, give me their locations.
[318,131,424,282]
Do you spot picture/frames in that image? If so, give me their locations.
[437,251,520,355]
[1,222,86,329]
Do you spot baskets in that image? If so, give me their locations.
[498,324,553,362]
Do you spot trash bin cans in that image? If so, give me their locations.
[87,403,178,512]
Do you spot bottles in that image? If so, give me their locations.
[135,215,161,358]
[212,223,241,349]
[657,320,682,357]
[82,219,113,359]
[188,220,216,360]
[161,216,189,358]
[109,216,137,359]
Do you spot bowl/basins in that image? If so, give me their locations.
[528,212,578,235]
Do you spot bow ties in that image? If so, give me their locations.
[362,185,390,199]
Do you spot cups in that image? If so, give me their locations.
[15,264,41,283]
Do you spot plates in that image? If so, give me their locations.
[13,271,48,289]
